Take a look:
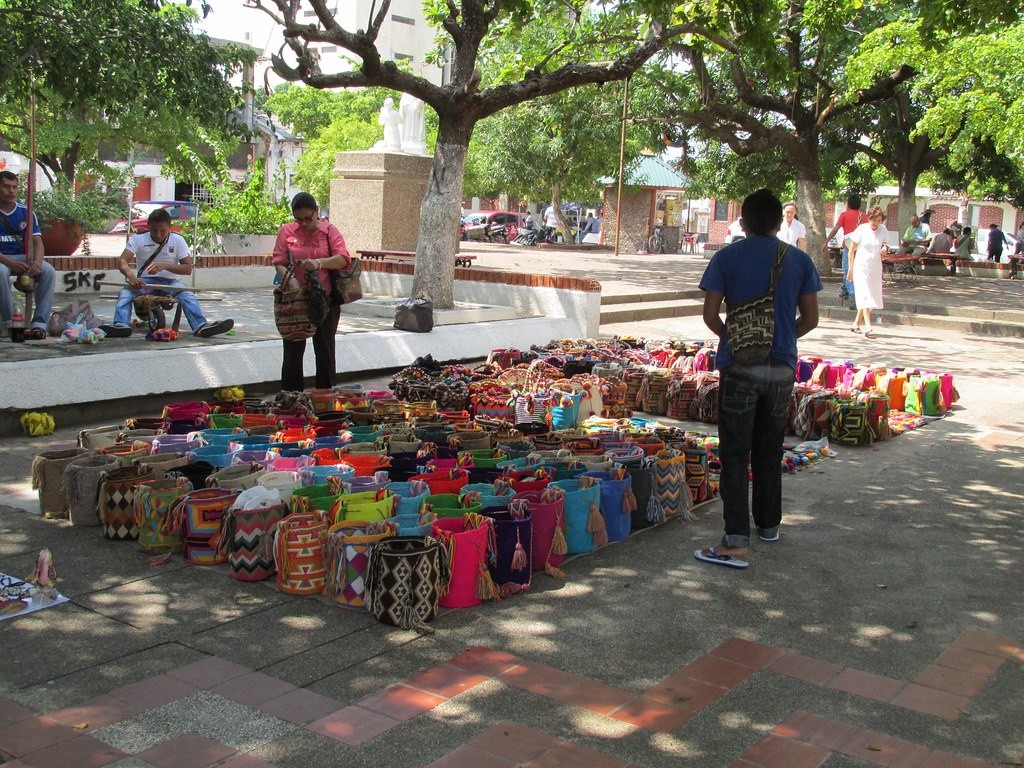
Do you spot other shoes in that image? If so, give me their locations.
[198,318,234,337]
[839,285,848,299]
[1010,273,1018,280]
[847,295,856,310]
[31,327,46,340]
[98,324,132,337]
[0,329,13,343]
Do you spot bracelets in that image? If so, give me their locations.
[317,259,321,269]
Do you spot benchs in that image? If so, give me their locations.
[357,249,476,268]
[97,295,222,332]
[880,250,959,287]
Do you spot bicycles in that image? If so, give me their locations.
[647,228,670,254]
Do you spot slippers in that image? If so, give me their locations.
[694,547,749,569]
[760,529,779,542]
[866,330,877,339]
[851,327,863,334]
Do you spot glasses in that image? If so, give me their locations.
[295,209,314,223]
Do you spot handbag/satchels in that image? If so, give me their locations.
[274,259,316,342]
[589,224,592,231]
[393,295,434,333]
[47,299,97,336]
[33,335,960,635]
[843,233,858,252]
[326,224,363,305]
[726,292,774,355]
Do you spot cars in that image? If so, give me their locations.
[108,201,203,237]
[725,218,748,244]
[465,210,524,243]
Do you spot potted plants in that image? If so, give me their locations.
[32,163,140,256]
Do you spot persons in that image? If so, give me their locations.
[543,201,604,243]
[821,195,868,309]
[931,228,953,253]
[694,191,825,568]
[955,227,974,260]
[0,171,56,339]
[777,201,806,252]
[273,193,352,392]
[526,212,533,230]
[844,206,889,339]
[1016,221,1024,254]
[98,209,233,338]
[542,206,603,244]
[898,215,932,273]
[986,224,1009,263]
[378,68,424,153]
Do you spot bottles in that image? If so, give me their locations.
[11,305,25,343]
[13,294,26,322]
